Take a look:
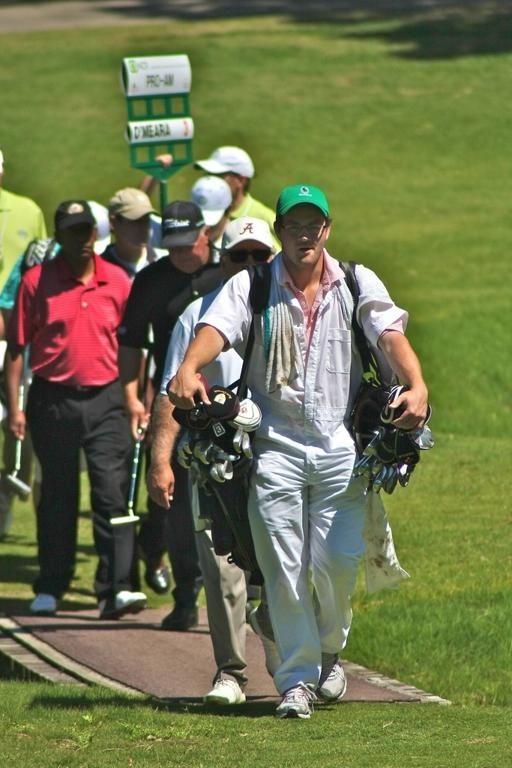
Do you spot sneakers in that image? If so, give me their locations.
[247,605,283,678]
[161,602,200,631]
[97,590,149,620]
[274,679,318,719]
[315,652,348,704]
[28,592,58,616]
[202,672,247,706]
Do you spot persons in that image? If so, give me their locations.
[116,202,220,630]
[137,153,177,246]
[167,185,428,719]
[148,218,281,707]
[192,145,283,262]
[0,201,111,510]
[3,200,147,621]
[190,175,233,262]
[96,188,172,594]
[1,149,48,542]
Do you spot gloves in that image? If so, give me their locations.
[349,371,437,495]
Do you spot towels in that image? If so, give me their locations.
[259,260,301,394]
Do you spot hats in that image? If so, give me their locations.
[275,183,332,222]
[86,199,114,255]
[192,145,256,179]
[218,215,275,256]
[107,187,161,223]
[158,199,207,250]
[189,174,234,227]
[52,199,96,232]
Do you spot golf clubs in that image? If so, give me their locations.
[174,384,263,481]
[7,351,35,500]
[353,388,437,495]
[109,348,155,528]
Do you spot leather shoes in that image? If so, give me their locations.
[134,536,172,595]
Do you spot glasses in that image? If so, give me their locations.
[225,246,271,264]
[279,221,327,234]
